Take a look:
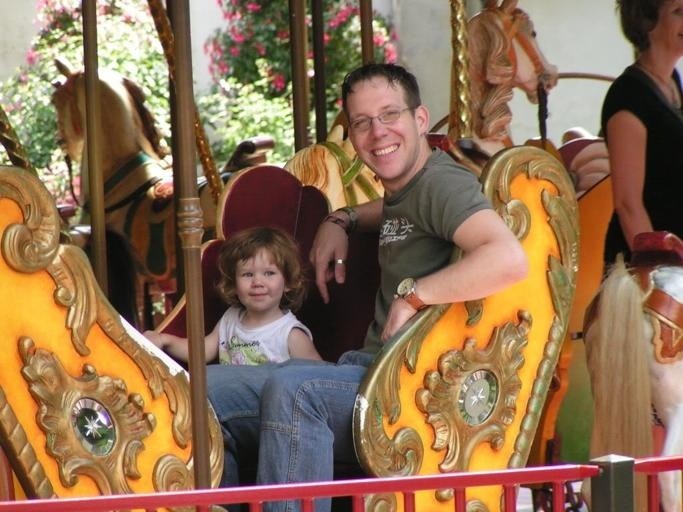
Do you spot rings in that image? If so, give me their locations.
[332,260,344,265]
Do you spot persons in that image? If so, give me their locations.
[602,0,682,288]
[206,62,530,511]
[134,224,336,366]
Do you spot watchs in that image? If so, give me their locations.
[395,276,425,312]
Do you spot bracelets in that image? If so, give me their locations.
[337,205,359,238]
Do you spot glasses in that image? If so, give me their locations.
[345,104,417,131]
